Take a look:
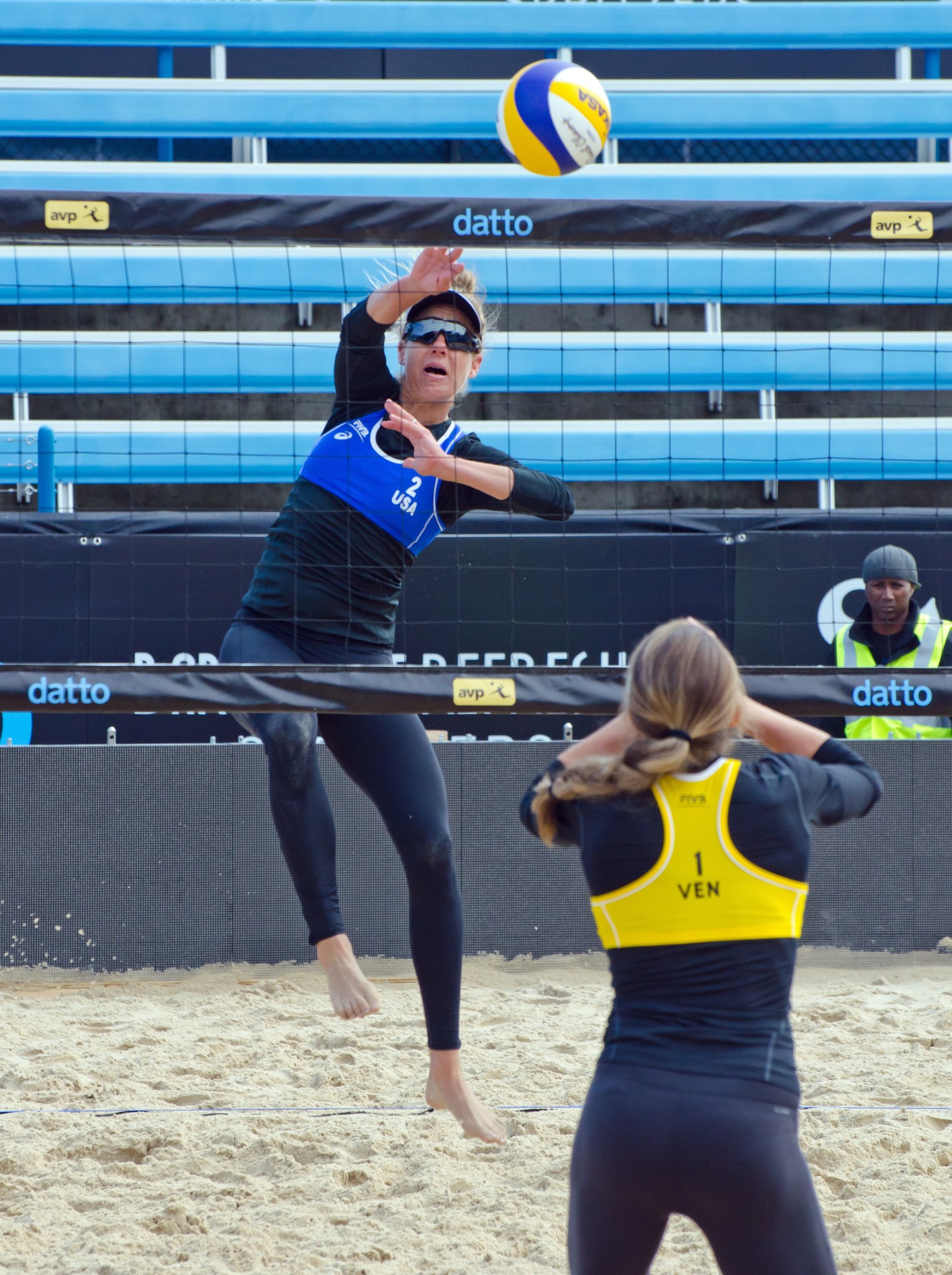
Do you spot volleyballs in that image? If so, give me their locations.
[495,57,614,177]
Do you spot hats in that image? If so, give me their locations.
[862,544,922,591]
[406,287,482,336]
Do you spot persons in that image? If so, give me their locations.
[220,244,573,1143]
[827,544,952,739]
[516,618,884,1275]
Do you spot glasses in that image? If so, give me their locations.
[400,316,482,354]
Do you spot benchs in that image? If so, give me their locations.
[0,0,952,510]
